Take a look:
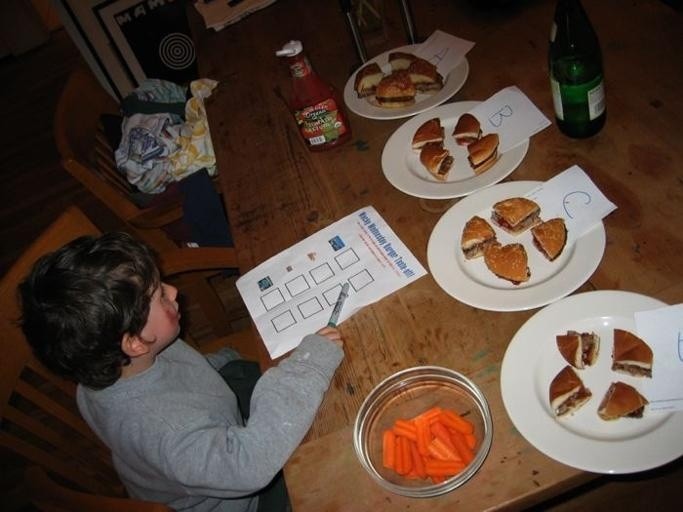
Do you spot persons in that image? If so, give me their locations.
[16,232,345,512]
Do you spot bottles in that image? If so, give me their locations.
[549,0,607,139]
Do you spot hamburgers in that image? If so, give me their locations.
[550,328,653,421]
[412,113,499,181]
[461,197,567,284]
[354,52,444,107]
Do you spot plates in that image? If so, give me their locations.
[381,99,531,200]
[499,289,683,473]
[426,180,606,312]
[343,45,469,120]
[274,39,352,151]
[352,366,493,498]
